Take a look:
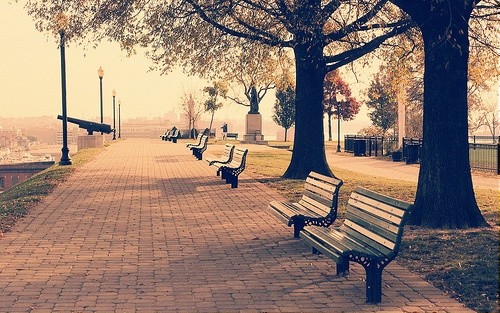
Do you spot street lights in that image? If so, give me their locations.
[112,88,117,140]
[97,66,105,135]
[117,100,121,138]
[56,18,74,166]
[335,91,343,153]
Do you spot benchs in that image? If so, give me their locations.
[207,144,236,176]
[186,133,204,155]
[227,133,239,140]
[160,130,180,143]
[268,172,343,238]
[213,147,248,187]
[189,135,208,160]
[300,186,415,303]
